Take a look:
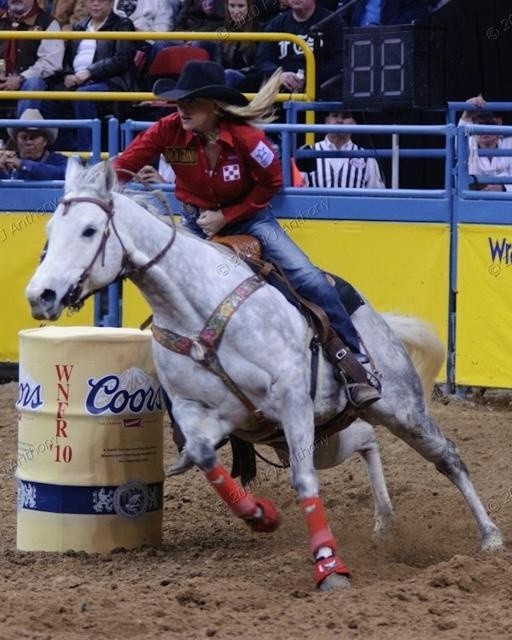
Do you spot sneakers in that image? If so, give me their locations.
[350,379,383,408]
[167,447,197,480]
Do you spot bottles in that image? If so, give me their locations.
[64,154,86,182]
[292,68,305,92]
[0,60,7,79]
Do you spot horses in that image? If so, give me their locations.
[26,154,504,592]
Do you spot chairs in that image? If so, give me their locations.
[130,45,209,108]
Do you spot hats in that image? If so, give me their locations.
[153,57,250,111]
[4,108,61,148]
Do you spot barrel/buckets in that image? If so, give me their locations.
[14,324,164,554]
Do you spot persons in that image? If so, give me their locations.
[455,95,511,194]
[1,1,446,191]
[115,57,381,454]
